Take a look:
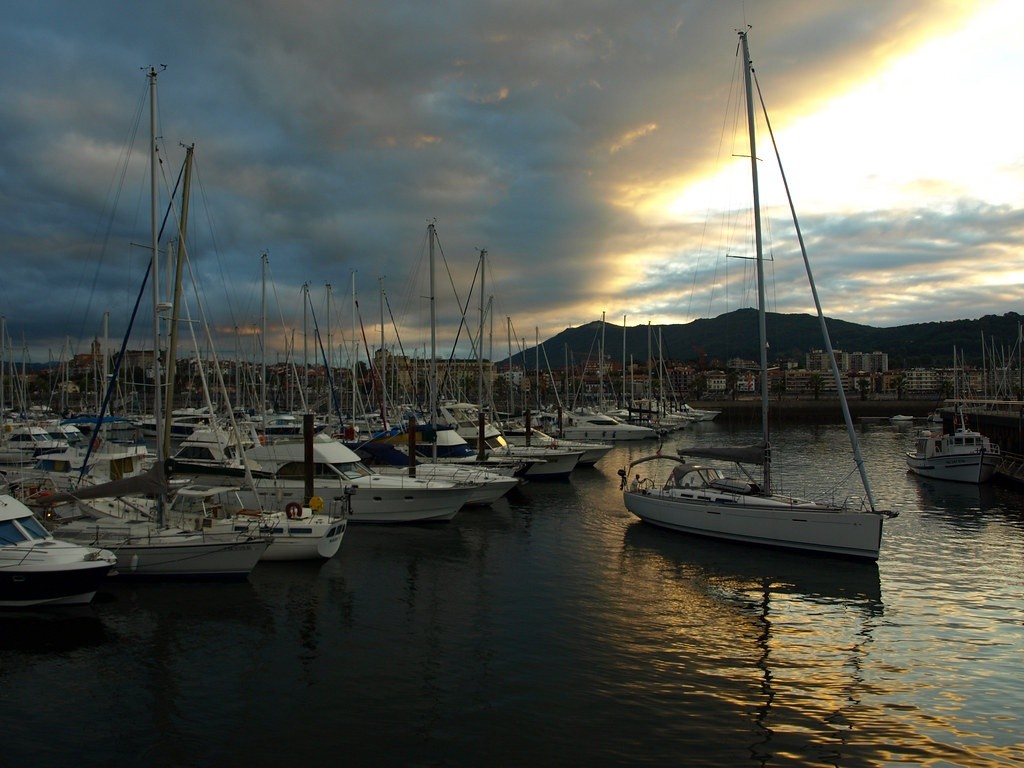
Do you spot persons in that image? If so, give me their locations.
[630,474,646,494]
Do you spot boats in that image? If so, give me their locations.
[992,450,1024,491]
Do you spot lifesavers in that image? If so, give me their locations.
[6,425,12,432]
[286,502,302,520]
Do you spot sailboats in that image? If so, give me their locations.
[616,23,901,559]
[905,321,1024,484]
[1,64,722,612]
